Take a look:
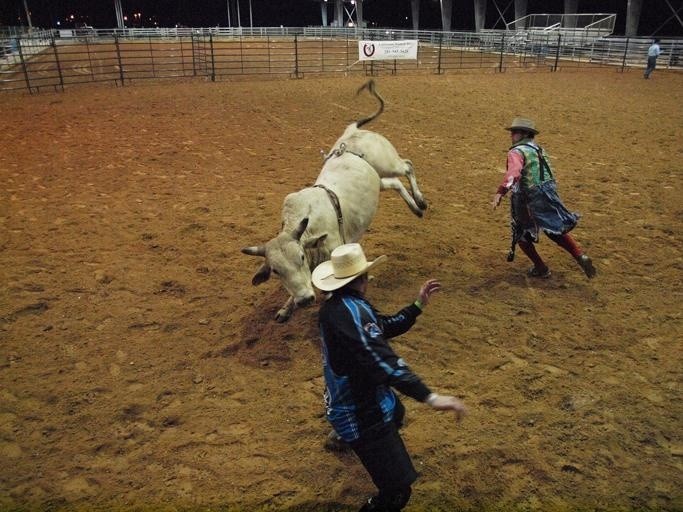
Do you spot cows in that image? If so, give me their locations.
[240,78,427,322]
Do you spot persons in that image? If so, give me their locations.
[312,243,467,512]
[644,38,664,78]
[492,117,596,279]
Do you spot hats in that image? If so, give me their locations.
[502,117,539,136]
[310,242,389,292]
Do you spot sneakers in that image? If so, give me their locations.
[526,266,551,278]
[643,74,650,79]
[575,253,596,280]
